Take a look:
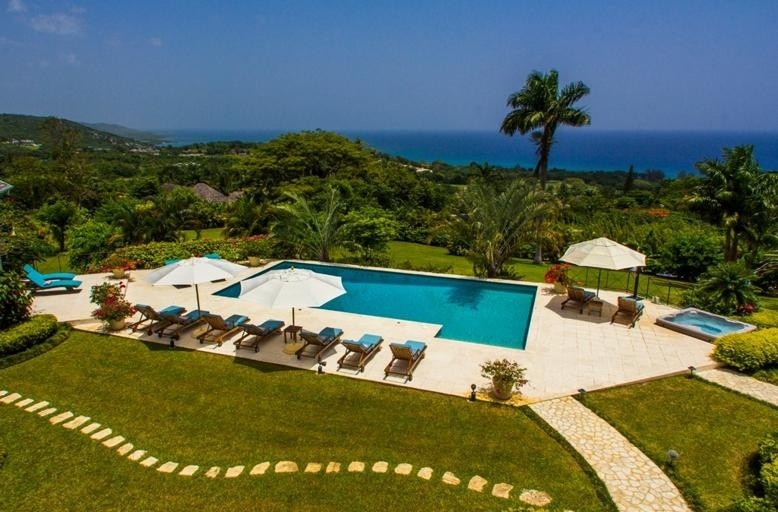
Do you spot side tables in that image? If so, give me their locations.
[284,325,303,344]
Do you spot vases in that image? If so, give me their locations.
[111,266,125,278]
[491,374,514,400]
[107,314,126,332]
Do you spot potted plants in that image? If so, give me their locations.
[246,247,263,266]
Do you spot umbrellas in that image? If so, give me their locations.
[238,266,347,340]
[143,254,248,318]
[557,237,648,298]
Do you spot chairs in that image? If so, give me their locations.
[558,279,644,328]
[336,333,384,374]
[165,259,178,264]
[204,254,220,259]
[22,263,75,282]
[155,310,210,340]
[231,319,285,354]
[293,326,342,366]
[196,311,250,346]
[26,270,82,296]
[131,300,186,335]
[383,340,428,383]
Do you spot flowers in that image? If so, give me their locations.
[88,278,135,323]
[478,353,528,395]
[100,252,137,273]
